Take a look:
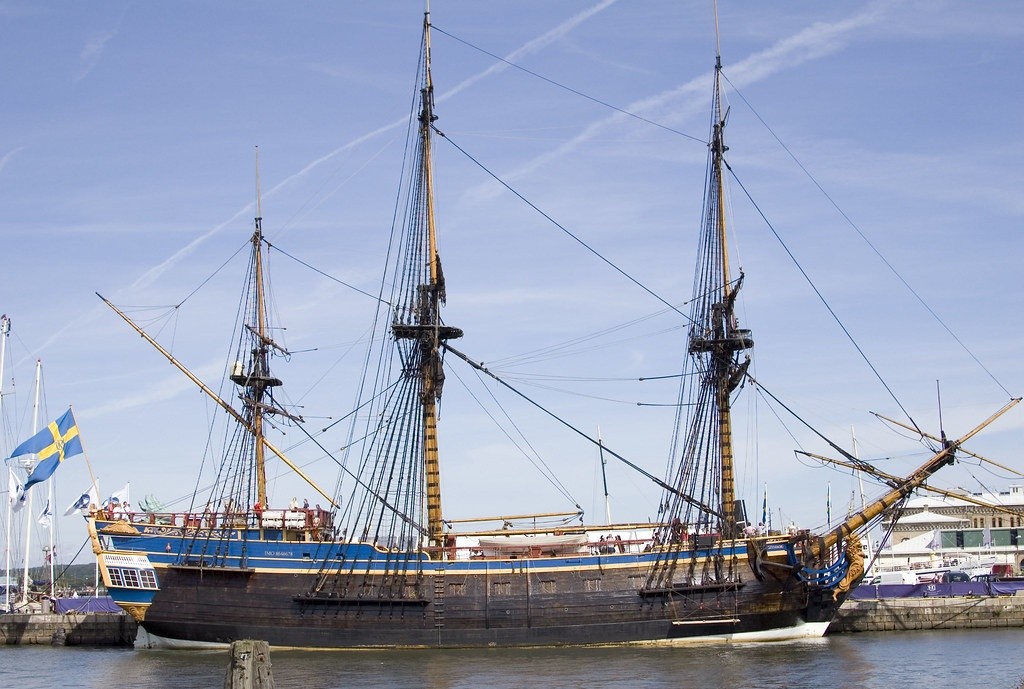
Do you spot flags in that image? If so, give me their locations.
[4,408,83,503]
[99,483,128,513]
[9,466,28,512]
[762,489,767,524]
[45,545,57,563]
[63,478,97,516]
[37,480,52,529]
[827,487,830,525]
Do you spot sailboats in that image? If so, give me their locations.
[0,2,1024,650]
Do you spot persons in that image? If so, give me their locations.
[99,501,133,522]
[204,495,310,526]
[598,520,799,555]
[55,587,78,598]
